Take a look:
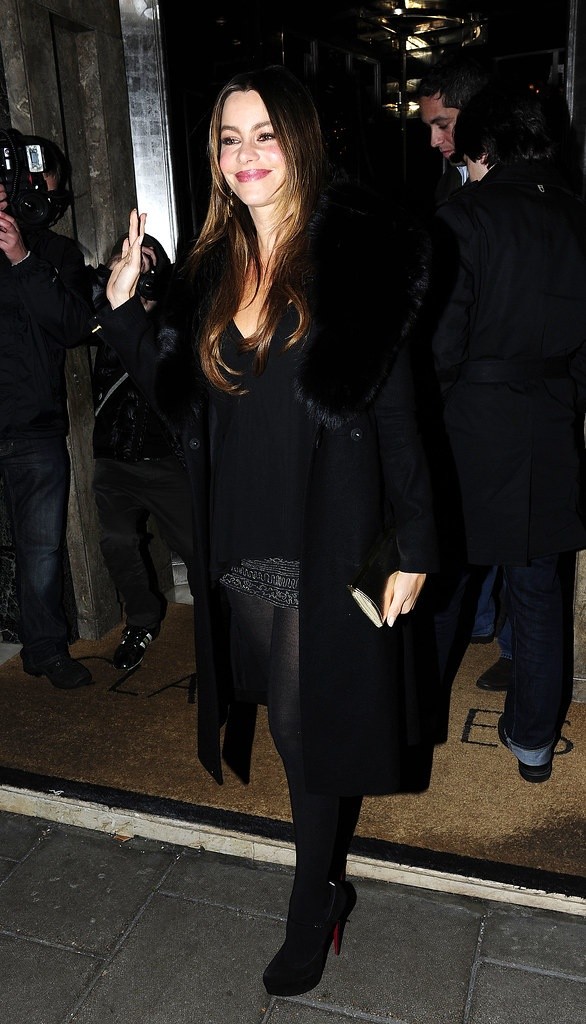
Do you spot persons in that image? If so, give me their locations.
[417,56,586,782]
[0,135,95,690]
[101,64,439,995]
[92,232,193,671]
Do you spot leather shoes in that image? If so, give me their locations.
[469,633,493,644]
[498,714,553,783]
[476,658,512,691]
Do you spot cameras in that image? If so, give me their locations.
[0,138,57,227]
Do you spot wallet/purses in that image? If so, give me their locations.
[346,534,396,628]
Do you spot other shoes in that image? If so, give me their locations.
[22,650,93,691]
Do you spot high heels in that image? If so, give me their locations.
[263,881,345,996]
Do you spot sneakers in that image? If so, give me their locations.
[114,623,162,670]
